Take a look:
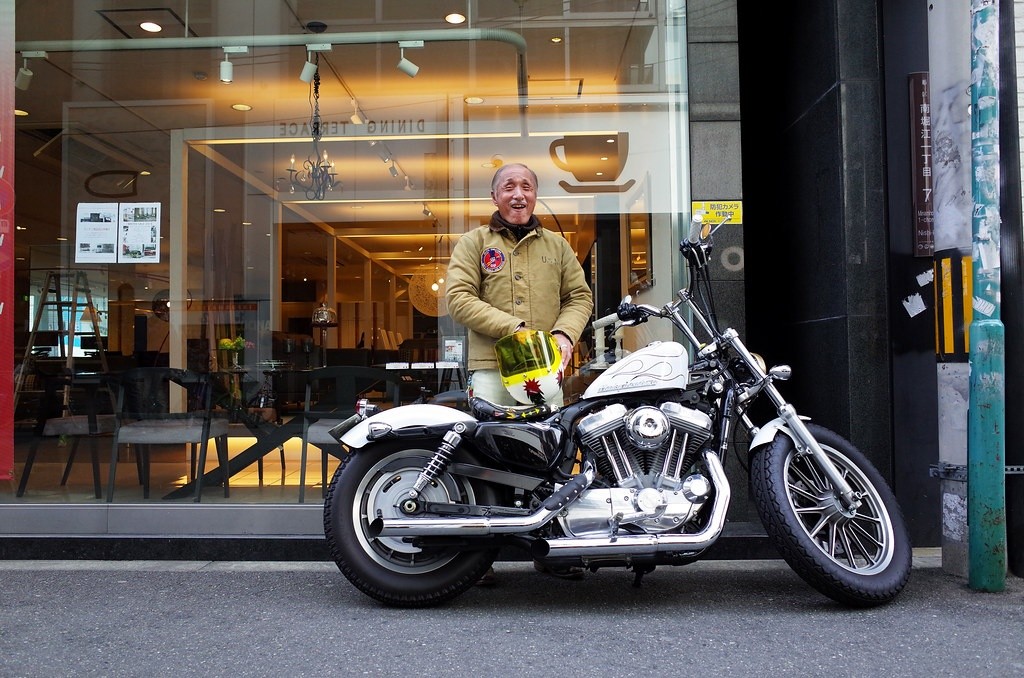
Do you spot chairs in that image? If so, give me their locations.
[15,356,404,503]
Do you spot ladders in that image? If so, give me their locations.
[14,269,132,447]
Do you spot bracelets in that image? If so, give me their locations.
[514,324,521,332]
[561,331,574,353]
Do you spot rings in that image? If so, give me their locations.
[560,344,568,351]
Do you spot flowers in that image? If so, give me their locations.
[220,334,256,352]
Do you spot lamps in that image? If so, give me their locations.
[389,165,399,178]
[370,140,393,163]
[407,233,449,317]
[273,21,347,202]
[311,303,338,371]
[220,46,249,85]
[299,42,332,84]
[396,40,425,78]
[15,51,49,90]
[423,201,431,217]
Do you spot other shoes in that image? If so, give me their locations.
[475,566,496,585]
[534,559,584,577]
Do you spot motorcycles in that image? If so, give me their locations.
[322,214,912,611]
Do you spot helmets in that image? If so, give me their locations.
[494,331,565,405]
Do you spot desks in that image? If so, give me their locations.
[192,366,328,402]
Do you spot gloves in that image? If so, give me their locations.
[495,336,534,371]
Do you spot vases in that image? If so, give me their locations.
[232,350,239,371]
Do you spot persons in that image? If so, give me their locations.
[445,164,594,586]
